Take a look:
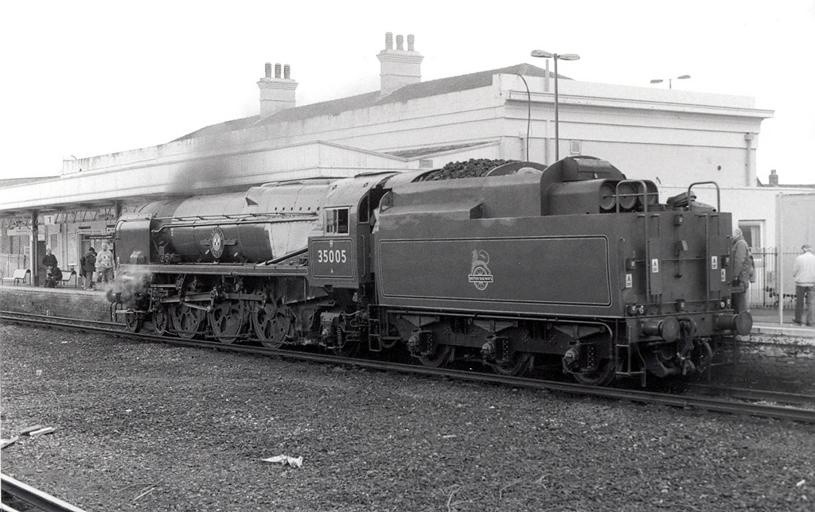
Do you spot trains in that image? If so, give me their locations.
[108,156,752,386]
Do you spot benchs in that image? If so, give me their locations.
[2,269,30,287]
[57,270,77,288]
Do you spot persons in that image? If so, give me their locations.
[792,243,815,328]
[42,243,116,288]
[732,225,756,314]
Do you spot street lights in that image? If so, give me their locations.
[531,49,581,163]
[651,74,690,89]
